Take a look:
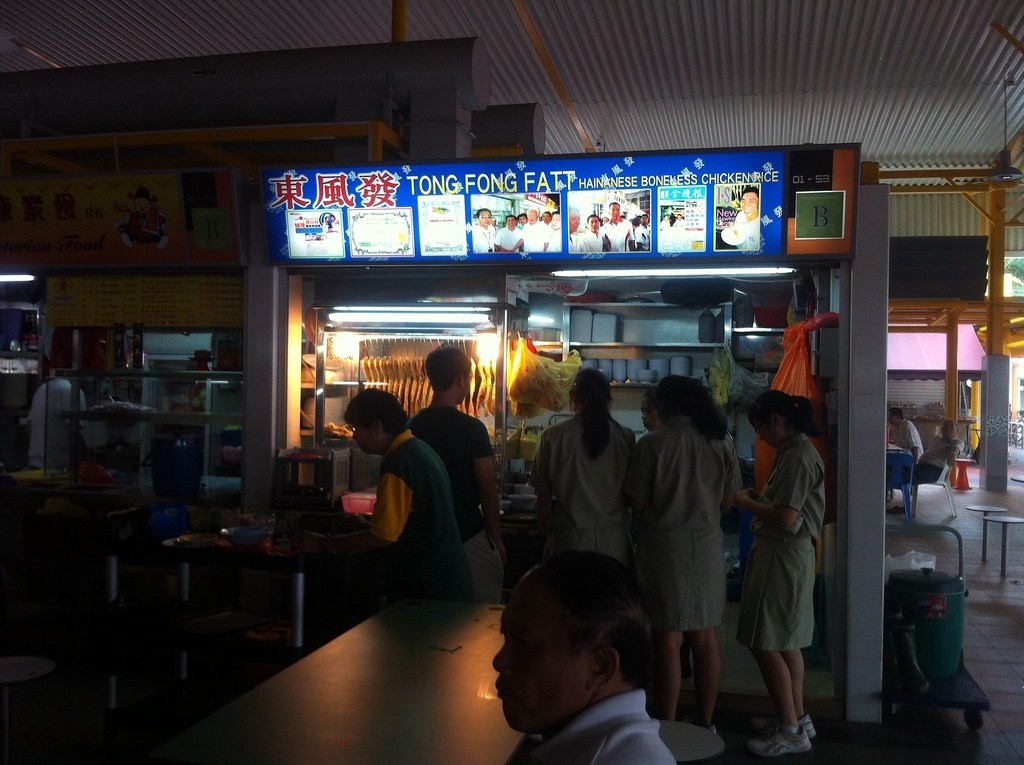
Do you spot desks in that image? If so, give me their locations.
[145,595,530,765]
[103,510,359,745]
[886,443,904,454]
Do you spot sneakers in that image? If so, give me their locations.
[744,722,813,757]
[761,714,817,738]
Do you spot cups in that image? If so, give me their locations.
[498,499,512,513]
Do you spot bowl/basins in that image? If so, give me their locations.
[751,304,791,328]
[507,495,539,511]
[580,357,692,384]
[226,528,271,545]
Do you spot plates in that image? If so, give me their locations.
[477,504,505,518]
[570,308,618,343]
[8,469,69,488]
[161,538,216,548]
[179,533,219,543]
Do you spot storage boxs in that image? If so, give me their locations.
[140,502,188,540]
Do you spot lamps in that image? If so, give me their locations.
[326,312,495,322]
[987,80,1024,180]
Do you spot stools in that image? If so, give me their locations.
[965,506,1007,561]
[0,655,56,765]
[982,515,1024,576]
[953,459,976,490]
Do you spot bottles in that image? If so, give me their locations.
[698,305,716,343]
[21,313,38,352]
[715,306,724,343]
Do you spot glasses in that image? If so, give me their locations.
[754,417,768,433]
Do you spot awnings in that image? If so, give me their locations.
[887,323,985,381]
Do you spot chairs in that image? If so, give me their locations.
[912,451,963,518]
[886,453,914,523]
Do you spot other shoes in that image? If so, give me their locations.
[681,714,716,736]
[886,505,905,514]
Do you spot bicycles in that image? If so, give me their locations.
[1012,410,1024,447]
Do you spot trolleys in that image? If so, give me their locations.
[889,520,994,734]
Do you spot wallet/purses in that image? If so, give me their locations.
[758,495,803,534]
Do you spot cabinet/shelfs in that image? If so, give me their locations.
[562,301,795,390]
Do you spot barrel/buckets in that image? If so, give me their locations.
[150,433,203,501]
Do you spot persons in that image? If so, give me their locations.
[472,208,562,254]
[734,187,759,249]
[26,374,87,469]
[530,369,636,568]
[662,214,684,228]
[641,388,666,432]
[888,422,964,514]
[888,407,923,465]
[493,551,679,765]
[288,389,473,607]
[569,201,650,252]
[406,347,507,601]
[626,375,743,733]
[735,389,827,757]
